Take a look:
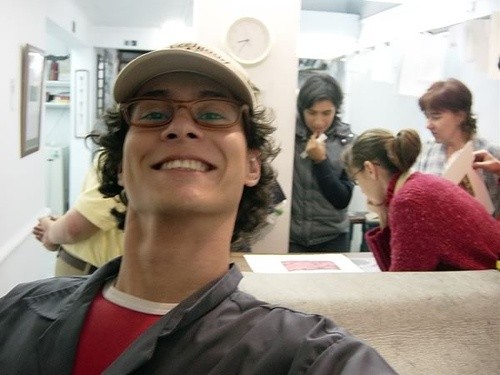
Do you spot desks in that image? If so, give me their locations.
[231,251,380,272]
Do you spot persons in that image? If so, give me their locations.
[346,128,500,272]
[33,147,127,277]
[472,150,500,176]
[288,74,357,252]
[0,40,399,375]
[411,78,500,219]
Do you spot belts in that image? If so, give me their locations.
[59,248,99,273]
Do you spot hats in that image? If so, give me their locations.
[112,43,256,114]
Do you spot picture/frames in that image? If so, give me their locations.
[21,43,45,157]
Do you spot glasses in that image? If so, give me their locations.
[119,95,255,131]
[348,165,364,186]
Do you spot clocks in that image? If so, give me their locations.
[227,16,271,65]
